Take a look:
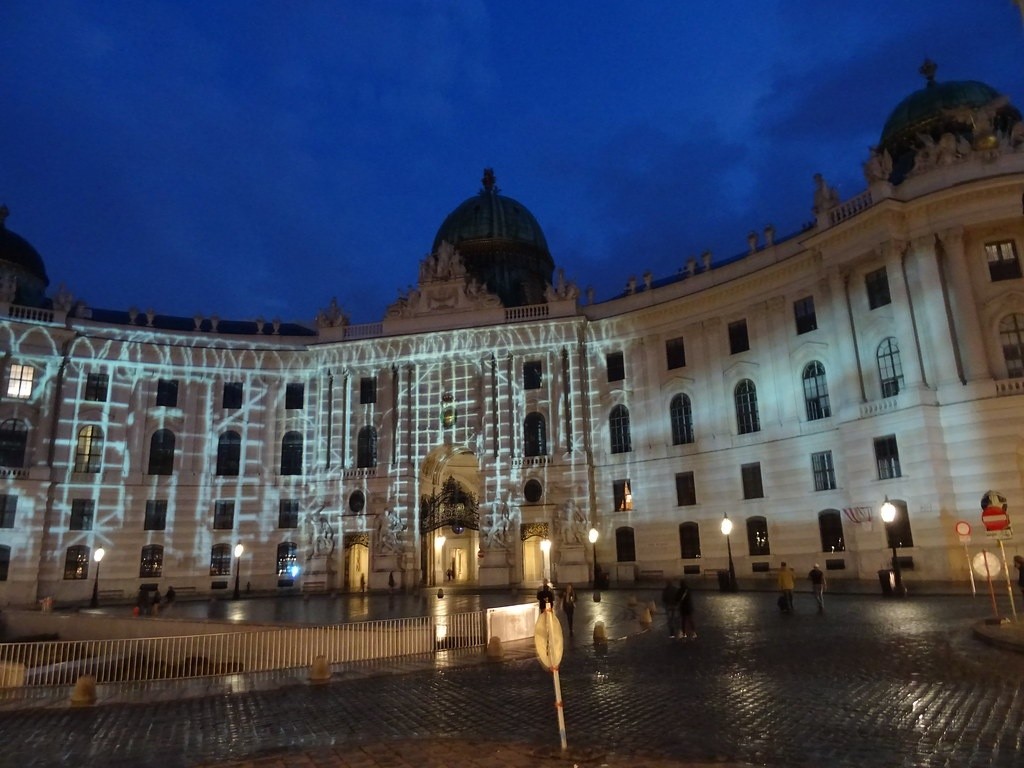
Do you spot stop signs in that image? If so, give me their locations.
[982,507,1008,530]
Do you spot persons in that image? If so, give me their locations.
[396,286,420,308]
[446,567,453,580]
[866,148,886,179]
[467,278,484,296]
[137,584,175,617]
[661,579,697,638]
[808,563,827,614]
[811,174,830,213]
[776,562,796,611]
[418,239,466,280]
[357,573,365,593]
[537,580,554,612]
[388,572,395,592]
[559,583,578,638]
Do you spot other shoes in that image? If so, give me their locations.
[691,633,698,639]
[668,636,675,640]
[682,633,688,638]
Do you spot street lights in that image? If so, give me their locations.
[880,495,905,598]
[720,512,738,592]
[233,541,243,600]
[91,544,105,609]
[589,524,599,590]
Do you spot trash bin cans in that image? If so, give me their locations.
[717,569,733,593]
[597,572,610,589]
[878,569,896,597]
